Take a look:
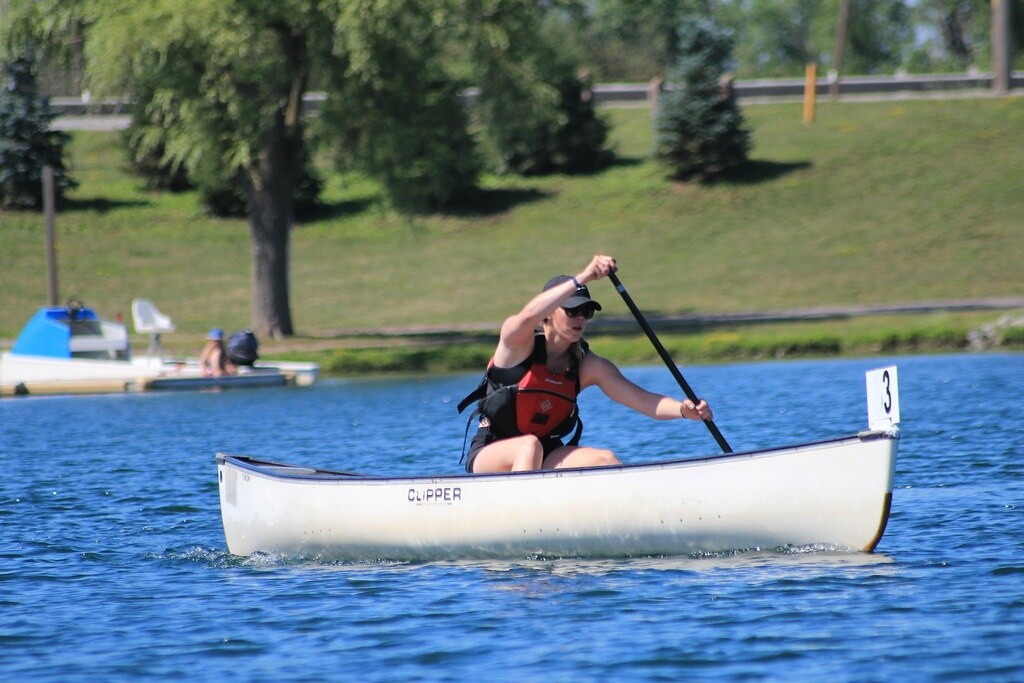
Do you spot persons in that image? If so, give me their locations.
[458,255,714,473]
[200,329,237,377]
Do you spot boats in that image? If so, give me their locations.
[215,367,901,562]
[0,166,317,395]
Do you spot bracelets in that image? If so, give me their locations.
[680,403,687,418]
[573,278,581,288]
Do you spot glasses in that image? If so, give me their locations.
[565,305,594,319]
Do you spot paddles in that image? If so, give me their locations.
[593,259,733,454]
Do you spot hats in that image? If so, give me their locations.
[543,274,602,311]
[206,329,225,341]
